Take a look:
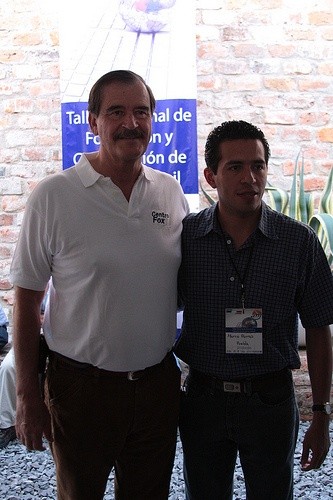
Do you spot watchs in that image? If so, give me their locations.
[312,402,333,414]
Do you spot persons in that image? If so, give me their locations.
[172,119,333,500]
[0,282,50,447]
[11,70,189,500]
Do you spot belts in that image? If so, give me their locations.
[190,368,290,397]
[48,353,175,382]
